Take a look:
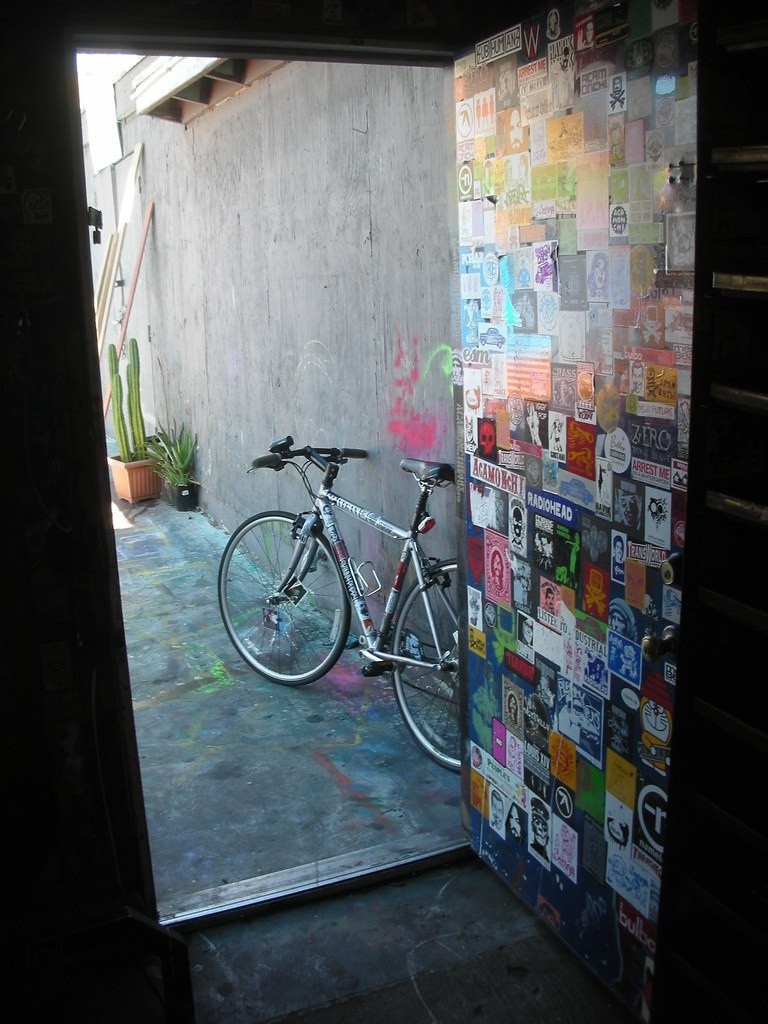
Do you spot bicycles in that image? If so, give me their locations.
[218,436,461,773]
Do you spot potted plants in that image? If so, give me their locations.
[133,418,198,512]
[107,338,163,504]
